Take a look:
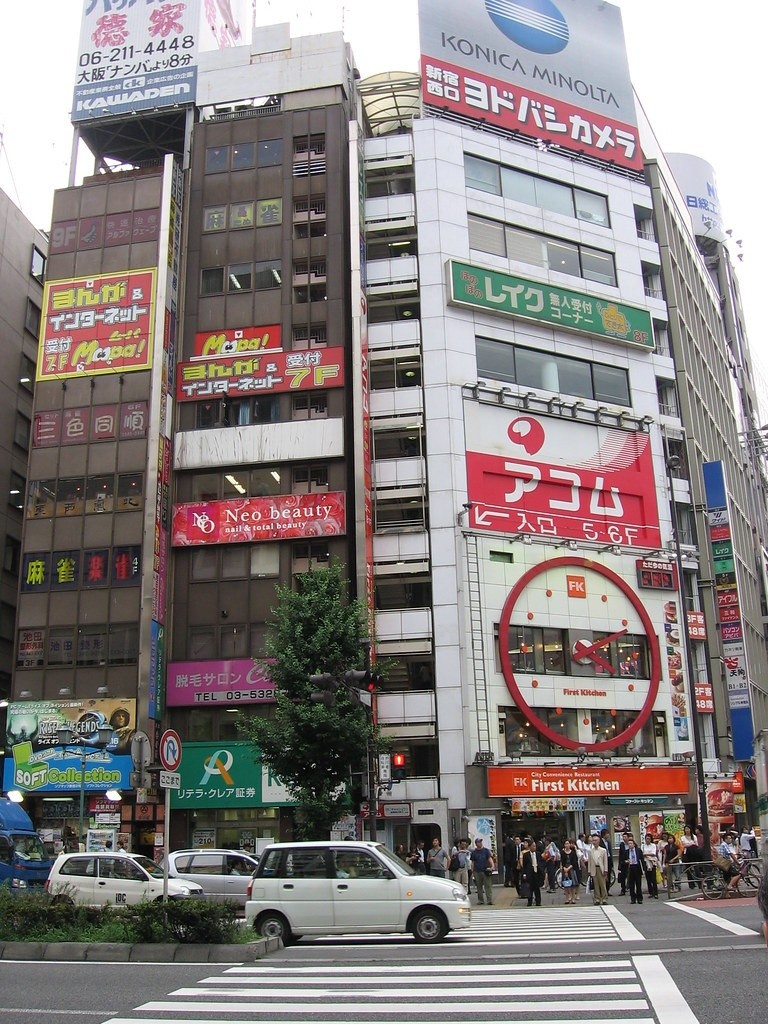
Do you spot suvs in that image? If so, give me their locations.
[157,849,275,909]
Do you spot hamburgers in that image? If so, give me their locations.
[704,782,734,823]
[644,815,663,842]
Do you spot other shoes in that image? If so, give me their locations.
[630,901,636,904]
[504,882,510,887]
[594,901,608,906]
[669,887,681,893]
[607,892,613,896]
[648,894,653,898]
[618,889,625,896]
[564,900,576,904]
[487,902,495,905]
[477,901,485,905]
[546,888,556,893]
[654,894,658,899]
[511,881,515,887]
[638,900,643,904]
[662,884,668,888]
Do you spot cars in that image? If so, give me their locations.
[44,850,205,910]
[244,840,471,947]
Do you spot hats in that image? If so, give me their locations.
[475,837,483,843]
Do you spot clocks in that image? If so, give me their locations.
[498,556,662,753]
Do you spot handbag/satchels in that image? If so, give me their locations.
[448,845,460,872]
[714,855,731,872]
[484,870,492,876]
[655,866,663,884]
[618,873,622,883]
[585,876,595,894]
[562,876,572,888]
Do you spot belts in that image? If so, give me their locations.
[595,865,600,867]
[742,849,753,852]
[459,867,465,868]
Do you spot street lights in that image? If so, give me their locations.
[666,455,717,892]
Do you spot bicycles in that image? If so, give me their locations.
[701,848,763,900]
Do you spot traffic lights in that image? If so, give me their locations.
[309,670,383,709]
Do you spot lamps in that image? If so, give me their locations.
[105,787,124,801]
[472,746,695,764]
[7,790,27,802]
[520,533,669,560]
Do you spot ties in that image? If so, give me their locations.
[518,844,520,860]
[532,851,537,873]
[631,849,635,864]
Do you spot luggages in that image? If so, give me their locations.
[520,880,530,899]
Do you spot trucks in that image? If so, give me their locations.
[0,799,53,895]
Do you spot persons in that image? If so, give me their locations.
[470,838,495,905]
[104,841,113,852]
[503,825,704,906]
[718,834,741,899]
[394,844,406,862]
[415,840,426,874]
[740,826,755,852]
[336,857,355,878]
[451,838,472,895]
[230,859,242,875]
[406,843,424,871]
[426,837,451,878]
[107,705,136,755]
[117,841,127,865]
